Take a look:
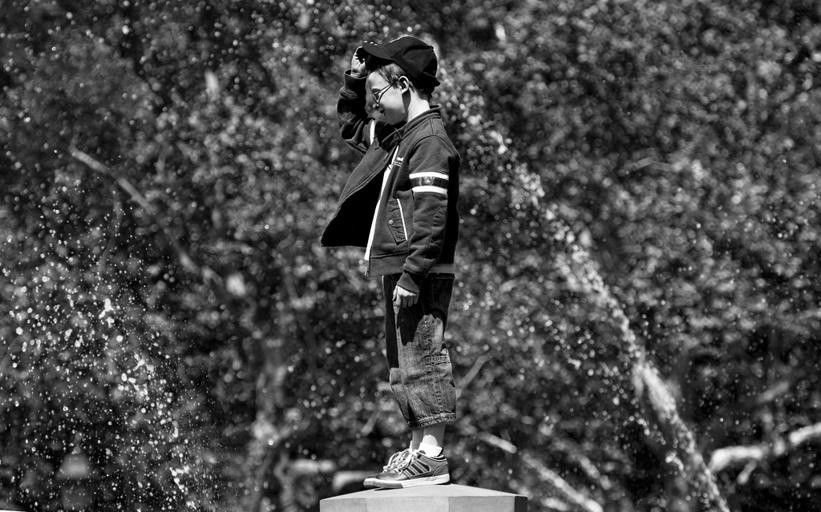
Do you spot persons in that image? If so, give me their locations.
[321,37,459,489]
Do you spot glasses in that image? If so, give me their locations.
[372,84,392,103]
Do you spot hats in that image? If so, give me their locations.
[362,36,440,96]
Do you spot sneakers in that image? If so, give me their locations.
[364,448,451,488]
